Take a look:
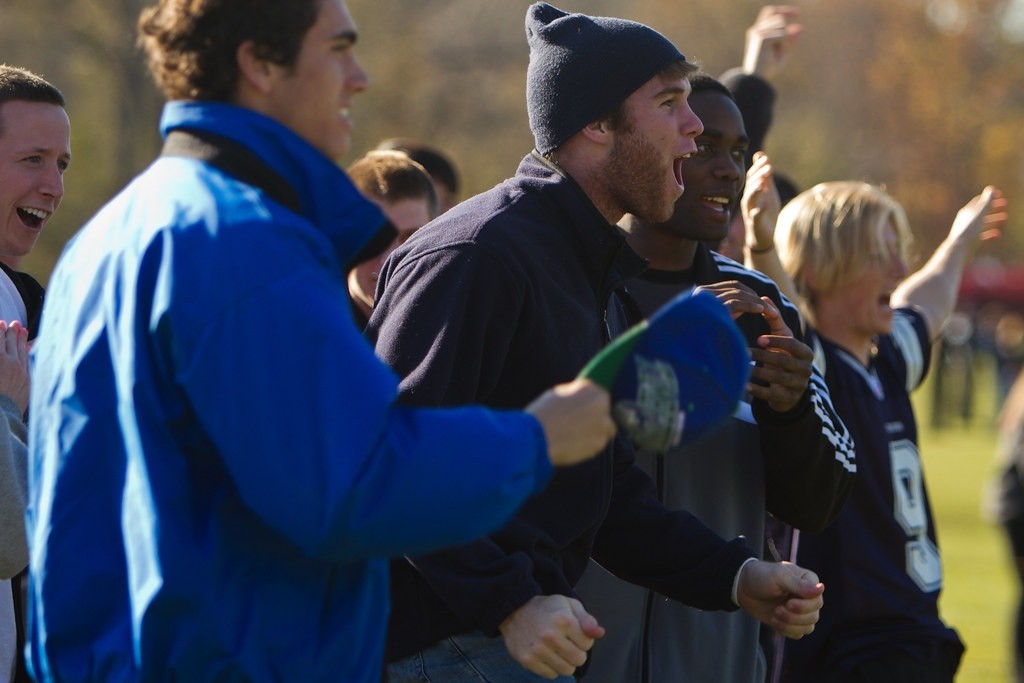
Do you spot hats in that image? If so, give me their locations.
[525,2,686,157]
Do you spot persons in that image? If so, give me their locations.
[0,316,31,580]
[377,137,461,215]
[581,0,1023,682]
[0,64,73,683]
[346,150,441,320]
[361,0,827,683]
[24,0,618,683]
